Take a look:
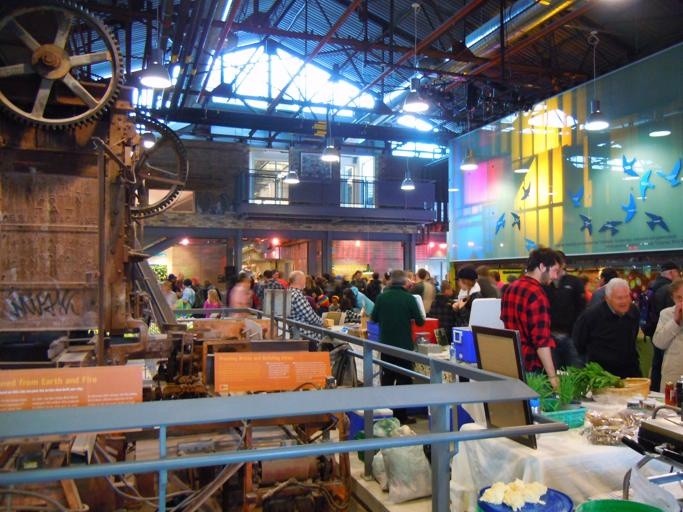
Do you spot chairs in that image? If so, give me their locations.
[48,337,88,368]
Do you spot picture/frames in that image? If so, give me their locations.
[299,152,334,184]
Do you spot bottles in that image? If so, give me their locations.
[676,375,683,409]
[663,380,675,406]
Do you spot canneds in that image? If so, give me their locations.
[665,382,677,405]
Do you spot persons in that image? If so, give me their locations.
[409,263,517,346]
[304,270,390,323]
[572,277,643,380]
[166,246,683,425]
[168,269,288,318]
[286,270,323,352]
[591,268,619,304]
[370,270,424,423]
[652,278,682,394]
[543,251,585,331]
[500,248,562,390]
[645,261,680,392]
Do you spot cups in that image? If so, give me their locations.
[529,399,540,437]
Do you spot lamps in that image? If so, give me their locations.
[320,112,340,162]
[140,0,173,87]
[400,159,415,191]
[403,2,429,112]
[583,31,609,130]
[282,132,300,184]
[459,113,479,171]
